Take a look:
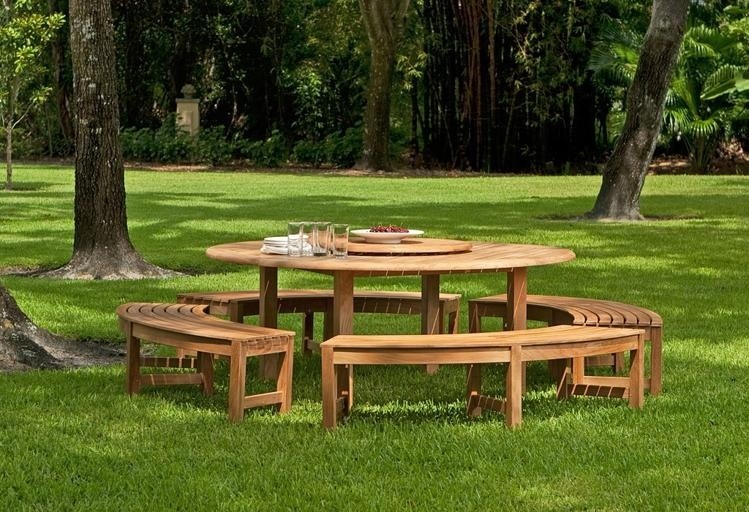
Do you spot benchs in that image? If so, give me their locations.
[178,289,460,356]
[320,325,645,425]
[115,301,295,420]
[468,294,663,395]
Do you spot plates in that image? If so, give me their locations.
[349,229,425,244]
[263,236,311,255]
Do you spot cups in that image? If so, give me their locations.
[288,221,349,258]
